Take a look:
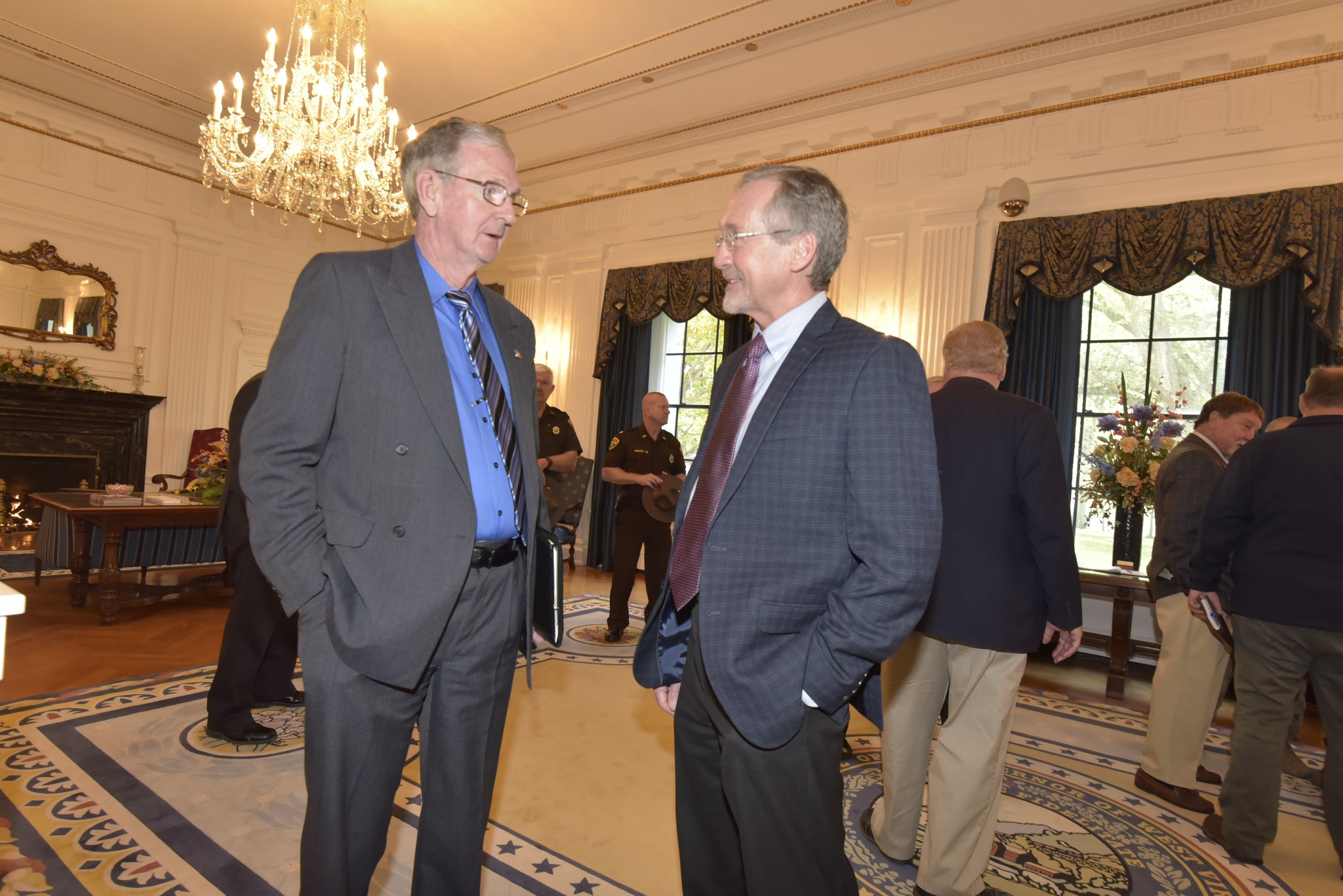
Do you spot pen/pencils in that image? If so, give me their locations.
[1210,613,1219,621]
[1126,569,1132,573]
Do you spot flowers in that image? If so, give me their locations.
[173,431,230,506]
[1072,368,1196,531]
[0,347,119,393]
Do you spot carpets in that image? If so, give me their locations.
[0,549,226,582]
[0,592,1343,896]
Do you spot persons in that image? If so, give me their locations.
[859,322,1082,896]
[1134,392,1265,815]
[633,163,943,896]
[533,363,584,534]
[1211,416,1314,772]
[205,371,304,744]
[1186,365,1343,870]
[238,116,533,896]
[600,392,687,643]
[921,376,944,725]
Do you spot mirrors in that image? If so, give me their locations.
[0,239,118,352]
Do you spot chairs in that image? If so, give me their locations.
[151,426,229,493]
[553,454,595,571]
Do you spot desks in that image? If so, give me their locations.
[1049,570,1161,701]
[29,492,220,626]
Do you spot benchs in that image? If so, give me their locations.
[34,505,225,587]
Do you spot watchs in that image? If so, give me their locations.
[545,457,553,469]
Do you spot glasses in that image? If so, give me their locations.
[716,228,791,251]
[434,170,529,216]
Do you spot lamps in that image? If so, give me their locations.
[197,0,418,239]
[996,177,1031,216]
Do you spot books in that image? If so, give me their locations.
[1197,594,1234,657]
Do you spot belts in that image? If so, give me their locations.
[470,539,519,568]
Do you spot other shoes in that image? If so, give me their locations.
[1282,742,1313,778]
[1203,813,1265,865]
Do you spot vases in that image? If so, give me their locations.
[1112,497,1144,572]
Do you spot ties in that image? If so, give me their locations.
[443,289,529,547]
[642,474,684,523]
[670,333,769,612]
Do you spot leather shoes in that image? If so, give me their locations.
[859,806,913,863]
[1196,764,1222,784]
[207,712,277,745]
[1134,768,1216,814]
[251,688,305,708]
[604,627,623,641]
[913,883,1013,896]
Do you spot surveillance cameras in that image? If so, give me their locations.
[999,177,1029,216]
